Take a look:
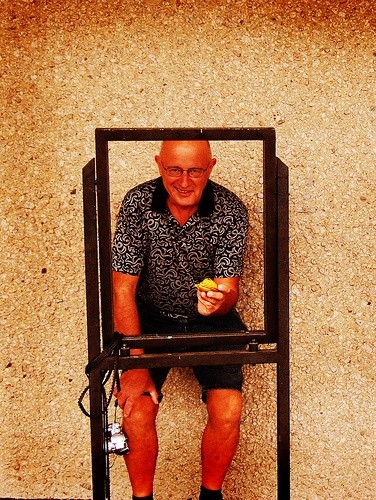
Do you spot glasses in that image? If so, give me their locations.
[159,161,210,178]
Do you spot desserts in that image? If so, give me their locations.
[195,277,218,292]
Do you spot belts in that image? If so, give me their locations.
[150,315,208,326]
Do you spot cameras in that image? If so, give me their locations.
[105,422,130,455]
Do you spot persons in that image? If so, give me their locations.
[110,140,253,500]
